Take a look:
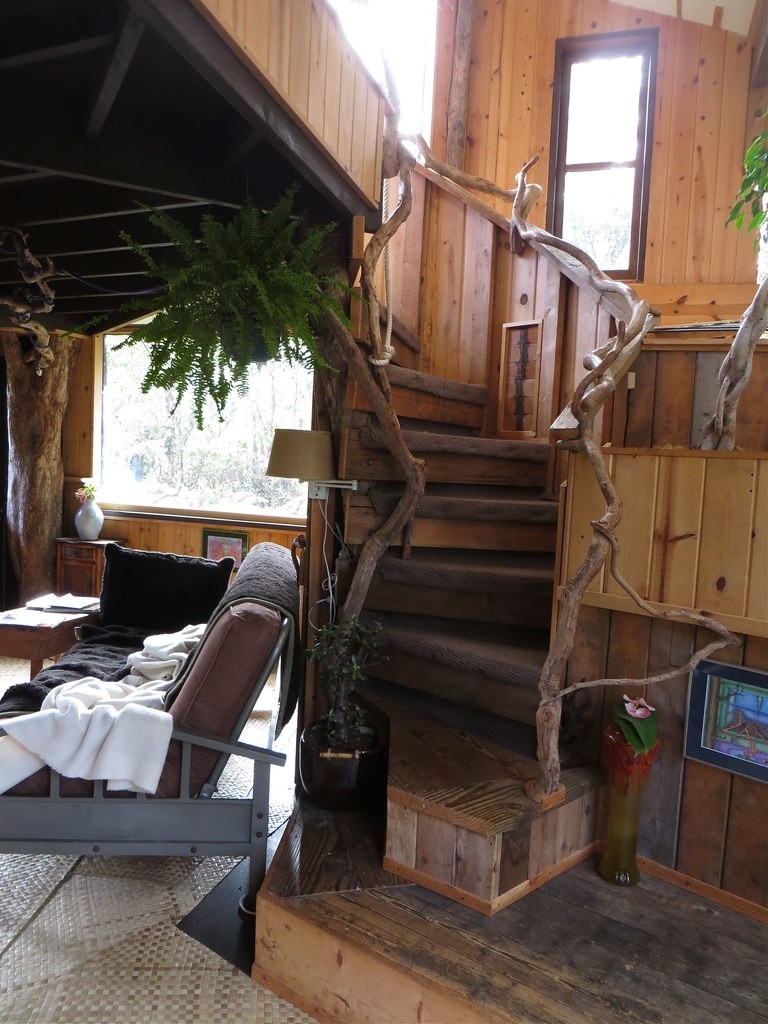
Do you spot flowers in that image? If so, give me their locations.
[613,695,658,756]
[74,483,99,503]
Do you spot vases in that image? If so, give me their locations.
[598,721,659,886]
[75,500,104,539]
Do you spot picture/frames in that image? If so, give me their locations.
[682,658,768,784]
[202,528,250,574]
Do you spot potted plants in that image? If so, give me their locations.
[299,613,390,810]
[49,185,369,432]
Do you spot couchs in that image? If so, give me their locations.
[0,540,297,915]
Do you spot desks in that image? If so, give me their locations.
[55,537,125,600]
[0,608,99,683]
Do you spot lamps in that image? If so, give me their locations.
[264,429,358,491]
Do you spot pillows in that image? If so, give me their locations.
[99,542,235,630]
